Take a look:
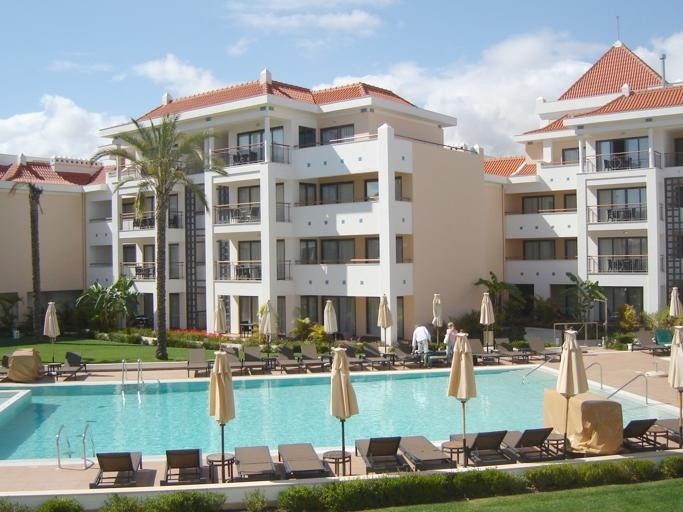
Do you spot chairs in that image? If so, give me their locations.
[89,451,143,487]
[134,264,154,279]
[603,157,641,271]
[219,342,449,375]
[55,364,86,381]
[160,427,564,487]
[653,329,673,352]
[624,419,658,451]
[239,320,254,339]
[227,262,260,279]
[132,215,177,229]
[187,349,208,376]
[655,418,679,442]
[634,329,667,356]
[216,204,259,224]
[0,358,8,380]
[467,337,562,365]
[130,314,152,329]
[229,150,257,163]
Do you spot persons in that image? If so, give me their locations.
[446,321,458,365]
[411,323,433,369]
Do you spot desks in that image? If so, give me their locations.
[46,363,61,377]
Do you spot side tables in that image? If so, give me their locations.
[207,360,215,372]
[644,425,669,450]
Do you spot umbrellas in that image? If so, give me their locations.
[377,294,393,354]
[324,299,339,348]
[669,286,683,319]
[259,299,278,367]
[480,292,496,354]
[667,326,683,449]
[556,329,589,461]
[432,293,444,346]
[330,347,360,476]
[209,351,236,484]
[214,298,227,351]
[43,301,60,372]
[448,332,477,468]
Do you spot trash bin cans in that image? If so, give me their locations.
[136,318,149,329]
